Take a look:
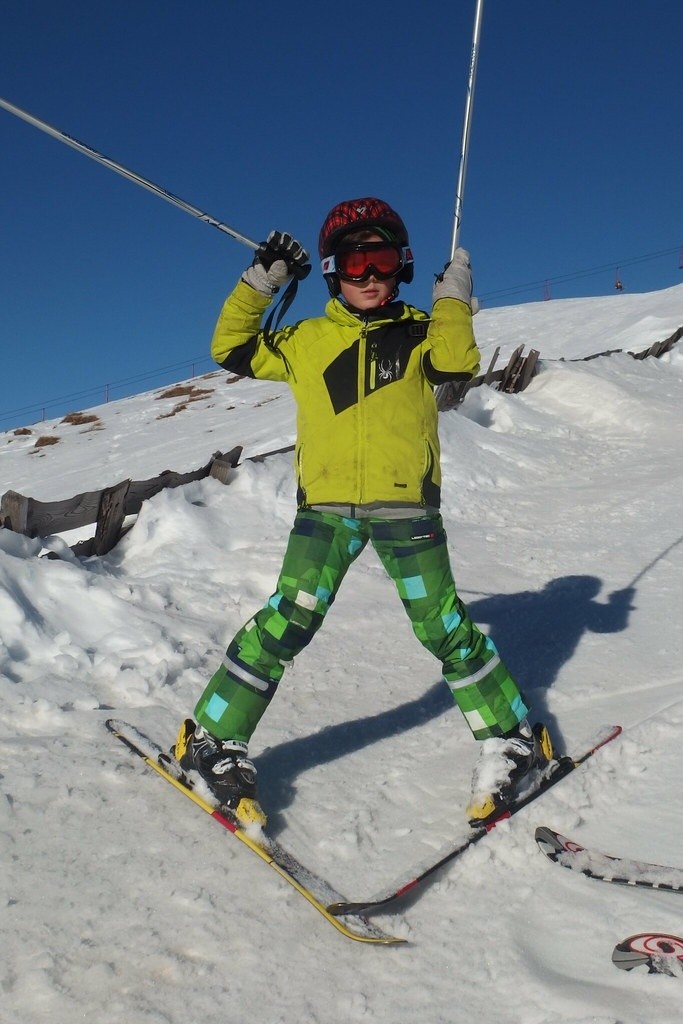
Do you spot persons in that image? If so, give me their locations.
[171,195,554,829]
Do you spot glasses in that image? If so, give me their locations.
[320,242,414,280]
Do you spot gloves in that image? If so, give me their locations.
[240,230,310,295]
[432,247,481,315]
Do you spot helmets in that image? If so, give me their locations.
[319,198,416,297]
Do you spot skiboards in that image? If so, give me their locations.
[534,825,683,979]
[109,718,625,945]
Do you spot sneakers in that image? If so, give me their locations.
[465,722,557,827]
[175,718,258,798]
